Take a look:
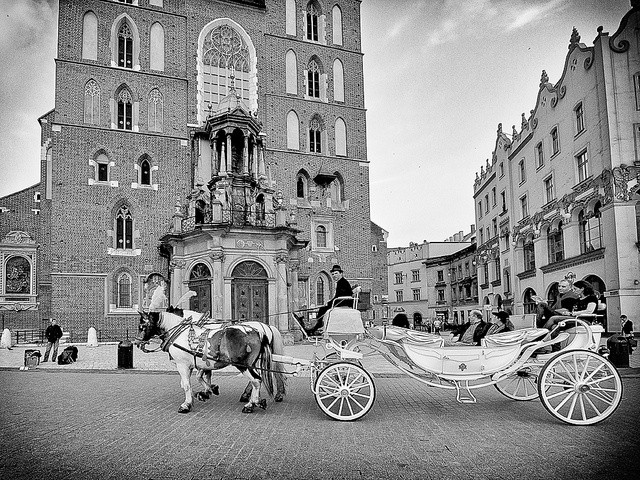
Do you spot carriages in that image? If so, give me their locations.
[135,286,623,426]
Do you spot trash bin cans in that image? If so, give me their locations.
[608,334,628,368]
[117,340,133,369]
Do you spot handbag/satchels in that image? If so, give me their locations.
[629,336,638,347]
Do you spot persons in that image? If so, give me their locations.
[529,279,579,342]
[304,265,354,335]
[620,315,633,355]
[543,281,598,354]
[447,310,487,345]
[471,311,512,346]
[186,183,207,229]
[42,319,63,362]
[426,317,444,335]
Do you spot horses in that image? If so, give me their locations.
[134,310,276,413]
[166,305,288,403]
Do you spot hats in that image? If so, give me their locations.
[491,310,509,320]
[257,173,267,181]
[329,264,344,274]
[194,181,204,186]
